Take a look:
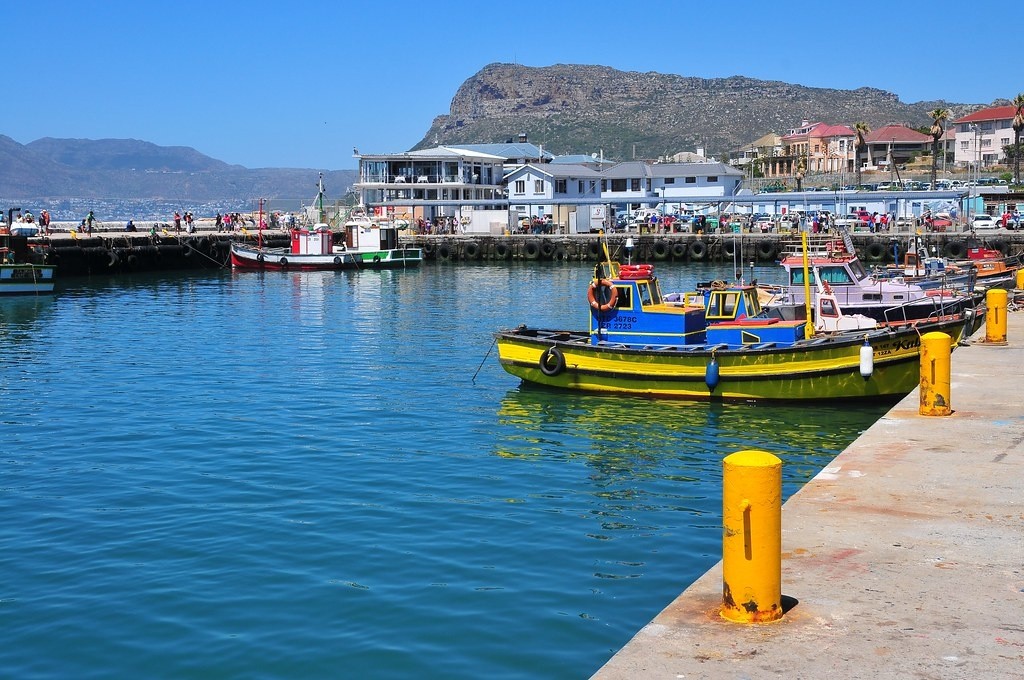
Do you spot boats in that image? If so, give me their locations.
[659,229,1024,335]
[229,198,366,272]
[313,170,423,269]
[0,234,59,295]
[492,260,971,403]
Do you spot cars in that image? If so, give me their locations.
[675,210,876,234]
[897,213,1004,233]
[792,177,1024,192]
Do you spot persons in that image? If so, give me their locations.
[967,265,978,295]
[262,212,298,234]
[1001,209,1021,232]
[6,251,16,264]
[919,207,976,233]
[150,226,161,244]
[860,211,898,234]
[0,210,55,236]
[77,210,97,234]
[718,210,842,235]
[416,215,467,235]
[510,214,553,235]
[214,211,255,233]
[645,213,713,235]
[173,210,198,235]
[126,217,137,232]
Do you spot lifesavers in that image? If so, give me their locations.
[586,279,618,311]
[671,243,685,257]
[944,242,965,259]
[687,241,707,260]
[617,264,655,274]
[256,253,264,261]
[372,255,381,264]
[333,257,342,265]
[987,239,1010,257]
[432,239,640,264]
[866,242,904,260]
[757,240,775,258]
[279,257,287,266]
[618,269,652,280]
[721,240,739,259]
[651,240,671,260]
